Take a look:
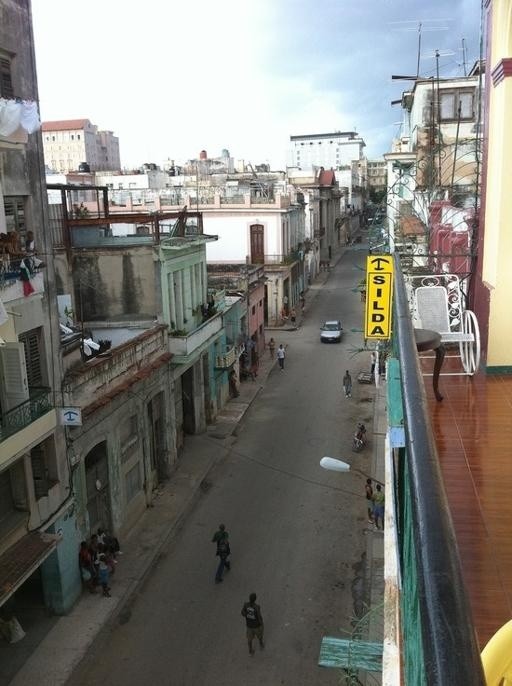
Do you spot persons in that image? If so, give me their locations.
[365,479,374,524]
[229,370,240,396]
[213,524,231,583]
[79,526,124,596]
[343,370,352,398]
[2,230,38,257]
[371,484,385,530]
[277,344,286,371]
[242,592,265,655]
[290,309,297,327]
[266,338,275,358]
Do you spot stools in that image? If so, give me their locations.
[413,328,447,402]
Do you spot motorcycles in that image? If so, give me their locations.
[352,422,367,452]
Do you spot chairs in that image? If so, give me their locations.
[403,273,481,378]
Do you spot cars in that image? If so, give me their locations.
[320,320,342,344]
